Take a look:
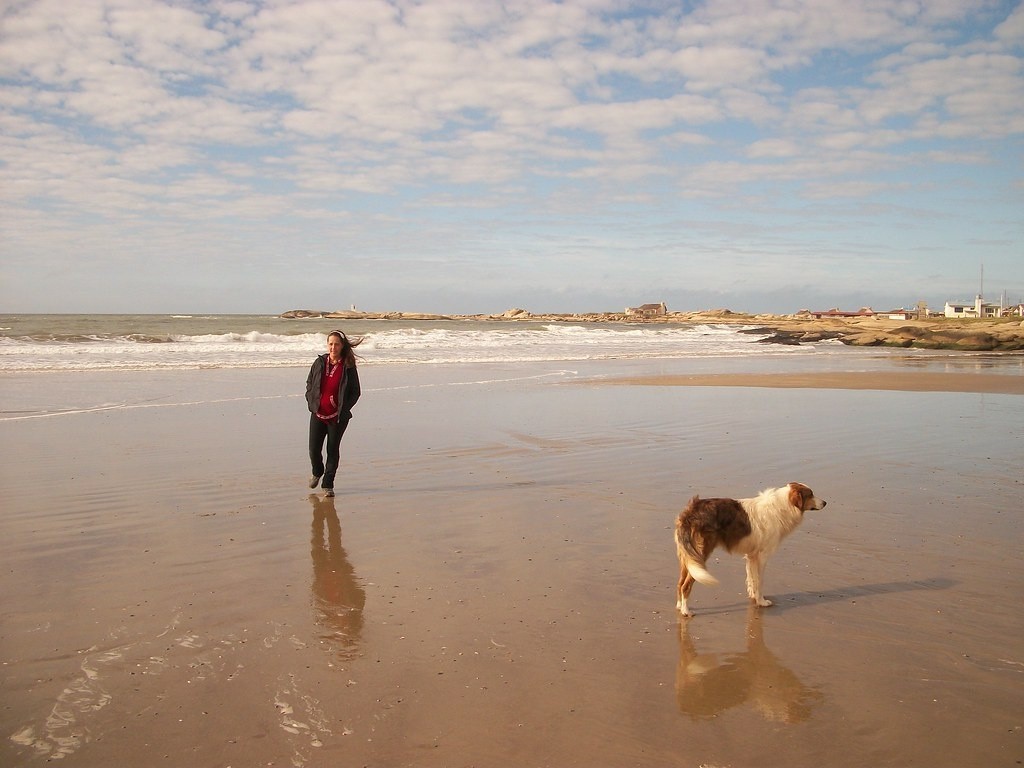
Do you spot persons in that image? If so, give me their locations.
[305,330,361,498]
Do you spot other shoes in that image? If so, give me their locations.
[322,488,334,497]
[309,475,319,489]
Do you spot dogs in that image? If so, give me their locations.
[672,477,828,617]
